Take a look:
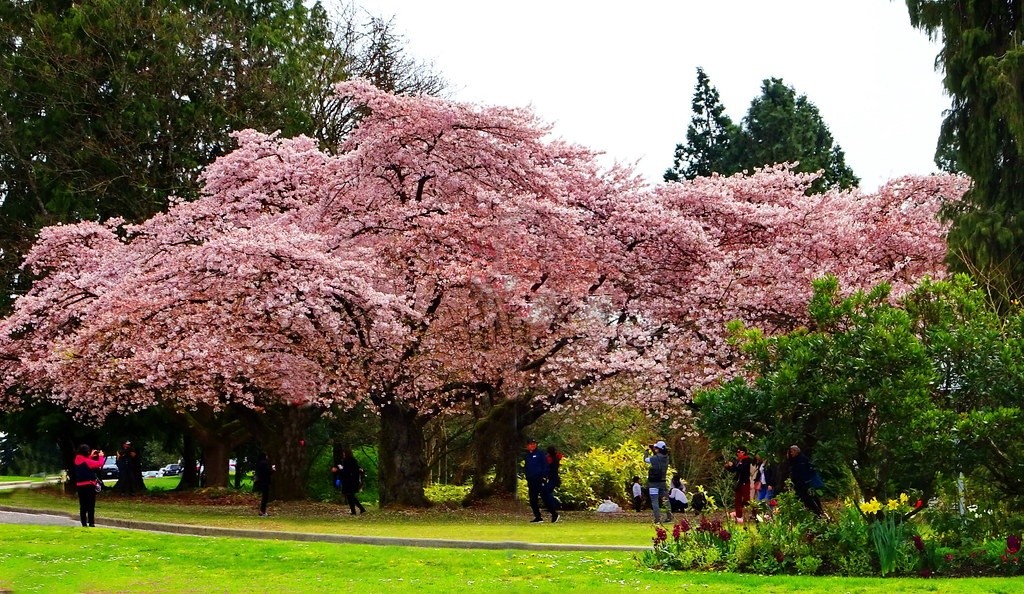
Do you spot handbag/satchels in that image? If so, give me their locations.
[95,483,101,492]
[754,481,761,490]
[730,480,739,491]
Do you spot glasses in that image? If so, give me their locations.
[737,451,741,453]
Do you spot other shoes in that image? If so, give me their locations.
[654,521,660,525]
[347,512,356,515]
[663,519,671,522]
[258,512,268,517]
[360,510,366,514]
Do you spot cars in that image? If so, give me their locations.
[100,456,119,479]
[142,464,182,477]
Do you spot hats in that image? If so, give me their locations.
[527,438,536,443]
[654,441,666,449]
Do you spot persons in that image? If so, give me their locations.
[667,478,688,513]
[633,477,643,513]
[524,437,560,524]
[74,444,104,527]
[690,487,707,515]
[195,459,204,486]
[643,441,672,526]
[252,452,277,518]
[723,445,823,522]
[544,444,563,510]
[670,475,686,493]
[116,441,137,496]
[337,449,365,516]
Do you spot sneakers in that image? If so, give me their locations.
[530,518,543,523]
[551,514,560,522]
